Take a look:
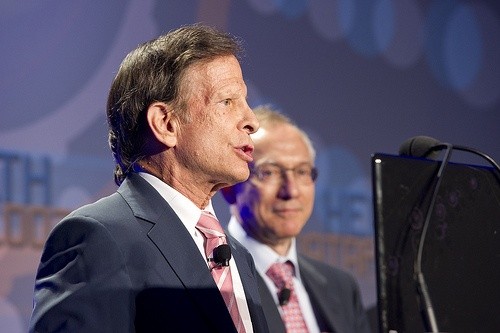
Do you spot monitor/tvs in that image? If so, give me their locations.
[371,153,500,333]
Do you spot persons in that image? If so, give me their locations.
[28,24,282,333]
[218,103,375,333]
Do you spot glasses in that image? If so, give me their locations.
[247,162,316,182]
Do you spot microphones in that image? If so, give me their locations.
[399,135,500,333]
[280,288,290,306]
[216,244,232,267]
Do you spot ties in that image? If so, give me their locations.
[265,263,310,333]
[196,212,246,333]
